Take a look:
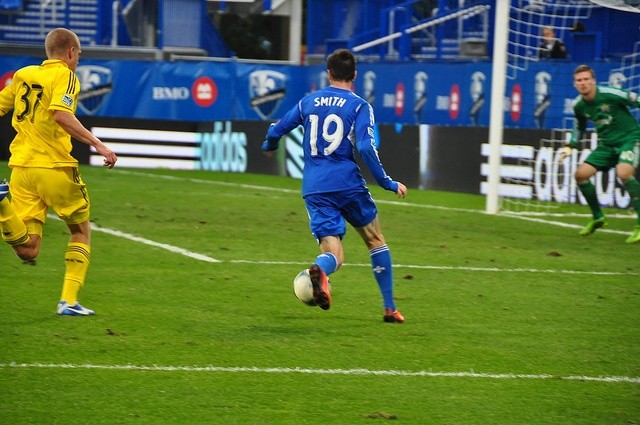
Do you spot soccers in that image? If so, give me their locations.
[293,269,331,306]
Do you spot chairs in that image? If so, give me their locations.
[323,16,381,59]
[535,30,600,63]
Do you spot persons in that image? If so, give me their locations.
[263,50,410,325]
[540,27,567,57]
[1,28,118,316]
[559,65,640,246]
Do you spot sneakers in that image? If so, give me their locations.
[56,300,95,316]
[578,216,608,236]
[309,264,332,310]
[384,308,405,323]
[624,224,640,243]
[0,181,9,201]
